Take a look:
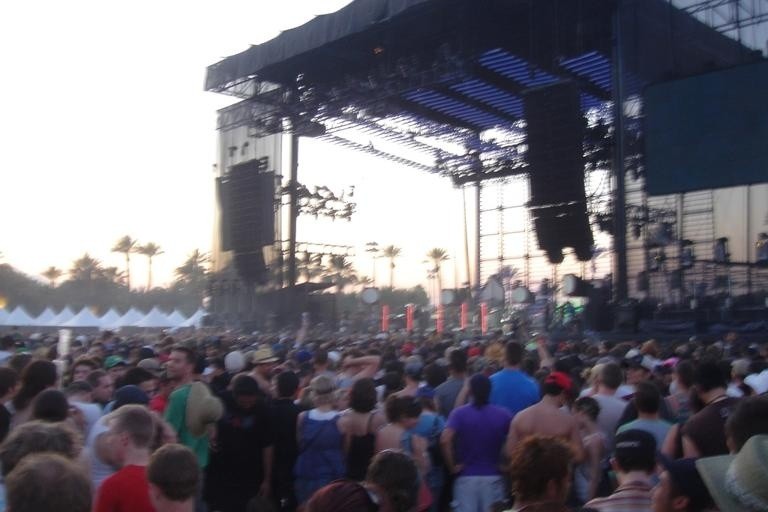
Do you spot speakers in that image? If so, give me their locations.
[216,156,276,285]
[525,82,594,264]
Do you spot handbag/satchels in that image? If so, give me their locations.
[573,470,590,504]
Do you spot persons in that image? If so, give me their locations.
[1,289,767,512]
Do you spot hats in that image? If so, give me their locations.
[115,384,150,406]
[225,350,247,374]
[621,349,653,371]
[105,355,126,369]
[545,373,573,391]
[252,347,279,365]
[295,350,313,363]
[309,376,338,403]
[695,434,768,511]
[183,381,224,436]
[401,342,415,353]
[483,344,504,358]
[416,388,435,398]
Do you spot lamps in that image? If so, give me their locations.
[439,285,465,307]
[561,272,593,300]
[510,286,535,304]
[355,284,378,306]
